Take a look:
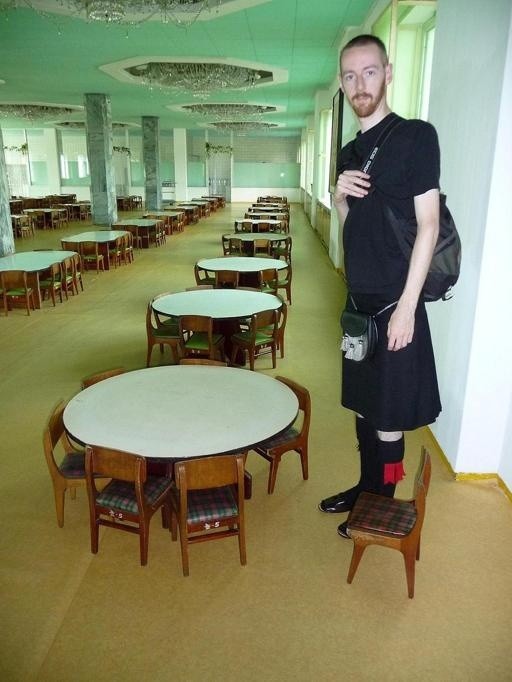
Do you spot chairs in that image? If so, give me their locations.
[342,445,434,599]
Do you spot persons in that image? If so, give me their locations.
[317,35,445,540]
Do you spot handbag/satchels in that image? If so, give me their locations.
[340,309,378,364]
[381,191,461,302]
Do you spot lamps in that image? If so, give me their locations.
[3,0,282,139]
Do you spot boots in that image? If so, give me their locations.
[319,413,375,515]
[337,432,406,539]
